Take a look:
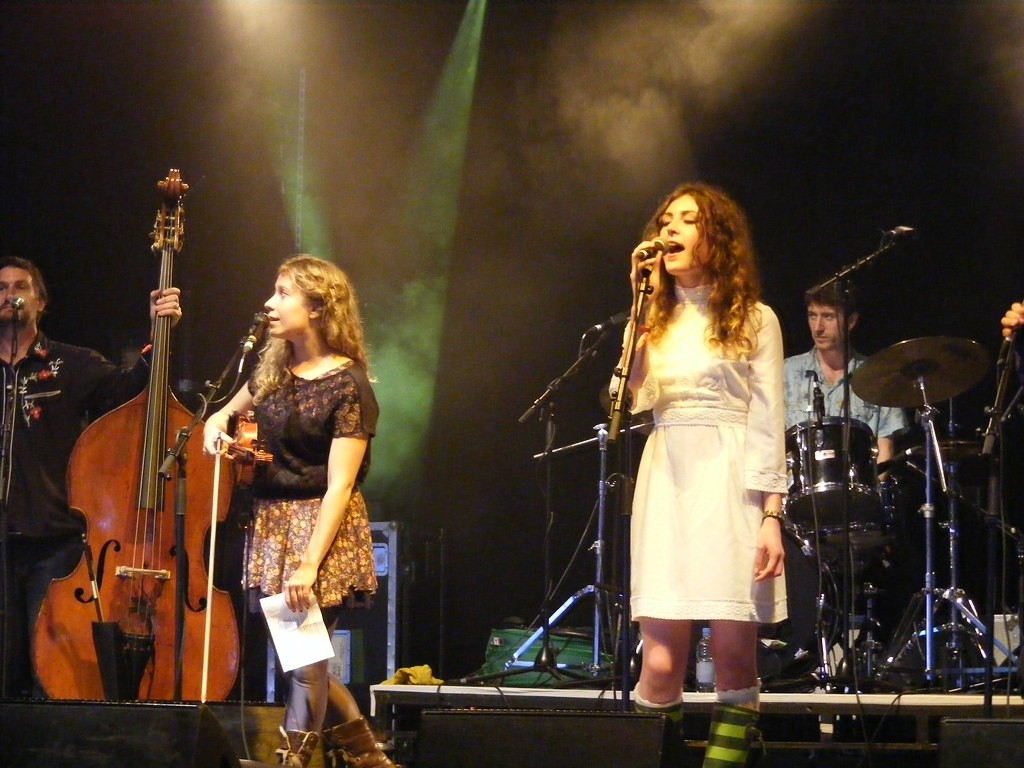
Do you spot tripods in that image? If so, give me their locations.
[863,380,1024,698]
[457,422,656,692]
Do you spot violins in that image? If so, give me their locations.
[230,410,273,485]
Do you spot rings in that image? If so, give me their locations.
[176,303,179,309]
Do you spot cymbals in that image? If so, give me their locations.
[853,338,990,408]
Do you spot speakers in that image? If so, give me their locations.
[939,718,1024,767]
[412,707,699,768]
[205,701,326,768]
[0,694,240,768]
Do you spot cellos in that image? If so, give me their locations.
[29,169,241,704]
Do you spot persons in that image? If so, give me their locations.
[610,183,907,768]
[202,255,399,768]
[0,257,185,700]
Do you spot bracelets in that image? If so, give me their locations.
[761,510,784,526]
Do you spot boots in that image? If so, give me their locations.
[633,683,684,768]
[274,726,318,768]
[320,715,397,768]
[700,702,759,768]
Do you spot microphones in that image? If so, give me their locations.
[586,311,630,332]
[10,295,27,308]
[242,312,271,353]
[634,236,670,258]
[811,378,826,417]
[885,226,918,239]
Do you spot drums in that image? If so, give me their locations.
[797,487,900,549]
[897,439,999,510]
[907,585,988,684]
[777,416,884,522]
[631,521,839,680]
